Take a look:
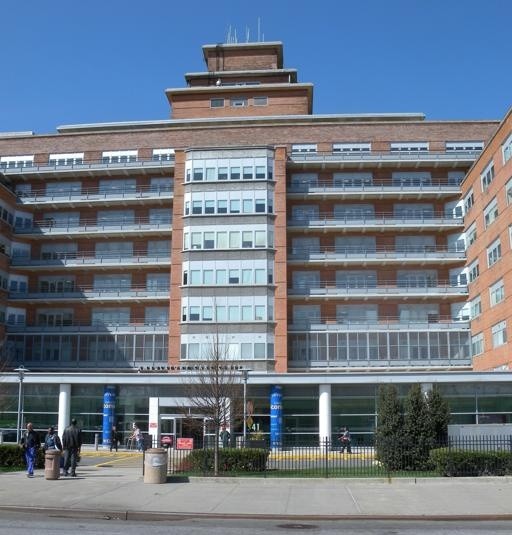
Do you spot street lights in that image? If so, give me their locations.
[14,365,30,445]
[238,369,254,448]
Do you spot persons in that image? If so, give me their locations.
[110,425,121,452]
[220,426,231,448]
[130,424,144,451]
[20,422,41,477]
[62,418,83,477]
[338,425,351,453]
[43,427,62,455]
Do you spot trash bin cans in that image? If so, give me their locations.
[144,448,168,484]
[44,450,60,479]
